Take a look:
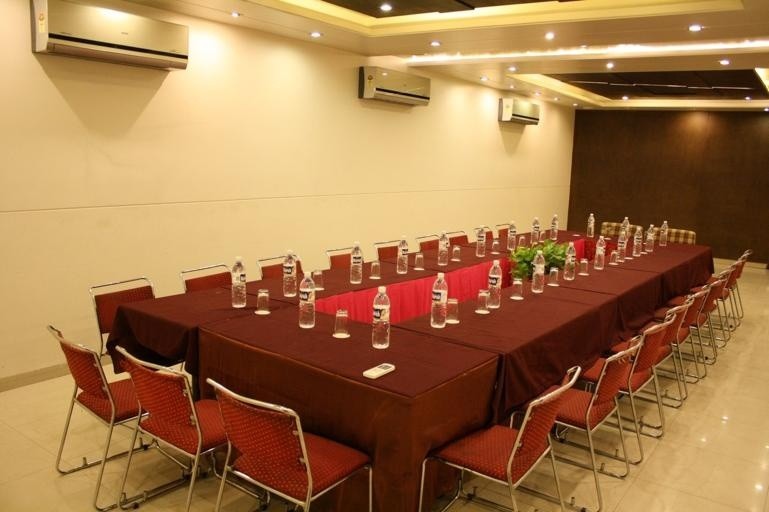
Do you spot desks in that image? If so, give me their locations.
[464,230,586,281]
[592,243,714,300]
[108,287,296,404]
[390,283,619,418]
[198,306,503,511]
[529,266,661,329]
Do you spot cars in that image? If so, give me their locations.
[579,257,588,276]
[510,278,524,300]
[446,298,460,326]
[608,248,618,266]
[333,309,349,341]
[369,231,547,280]
[548,267,559,286]
[256,288,270,316]
[313,269,324,292]
[476,289,490,315]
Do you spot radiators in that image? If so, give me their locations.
[362,363,395,381]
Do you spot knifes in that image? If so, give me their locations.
[499,98,541,126]
[32,0,189,70]
[357,64,432,106]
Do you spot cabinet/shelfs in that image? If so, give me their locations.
[587,211,669,266]
[531,250,546,294]
[429,272,448,328]
[231,256,247,308]
[593,236,606,270]
[283,250,298,297]
[486,260,504,309]
[563,241,577,281]
[297,273,316,330]
[349,214,559,286]
[371,286,390,351]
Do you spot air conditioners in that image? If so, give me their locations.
[357,64,432,106]
[499,98,541,126]
[32,0,189,70]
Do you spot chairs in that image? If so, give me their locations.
[48,325,186,510]
[115,345,269,512]
[583,313,683,439]
[44,220,515,356]
[608,303,690,429]
[654,250,751,399]
[418,365,581,511]
[510,335,643,510]
[587,223,697,262]
